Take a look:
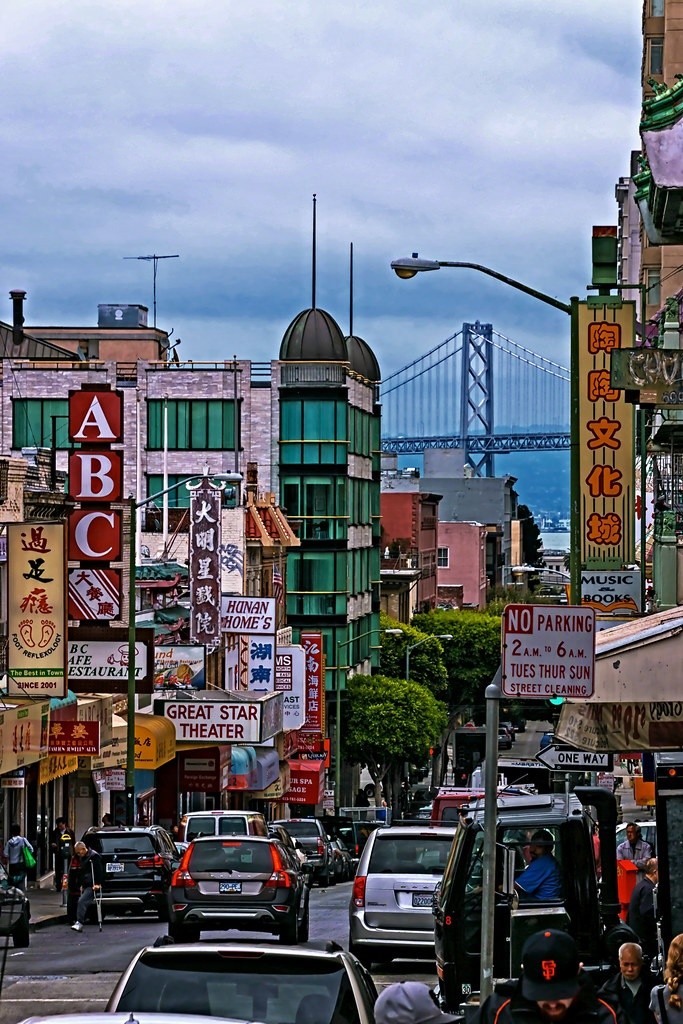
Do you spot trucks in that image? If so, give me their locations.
[432,751,683,1014]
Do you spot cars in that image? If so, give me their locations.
[167,832,315,945]
[350,824,458,970]
[265,825,302,869]
[498,728,513,749]
[105,934,379,1023]
[307,816,357,882]
[501,722,519,743]
[0,864,31,949]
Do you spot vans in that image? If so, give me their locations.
[435,758,552,850]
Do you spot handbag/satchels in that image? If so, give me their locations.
[22,845,36,867]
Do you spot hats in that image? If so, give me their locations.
[520,929,581,1000]
[373,981,465,1024]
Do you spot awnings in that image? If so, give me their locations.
[554,606,683,755]
[78,713,325,805]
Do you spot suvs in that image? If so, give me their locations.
[267,818,338,889]
[180,810,268,842]
[67,825,188,922]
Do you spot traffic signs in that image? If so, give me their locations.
[536,743,615,775]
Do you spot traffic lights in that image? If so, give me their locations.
[455,758,467,786]
[548,697,564,707]
[429,744,441,757]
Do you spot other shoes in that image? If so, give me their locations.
[71,920,83,932]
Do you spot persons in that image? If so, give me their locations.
[373,819,683,1024]
[4,813,148,933]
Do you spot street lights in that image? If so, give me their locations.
[125,470,245,829]
[389,251,580,605]
